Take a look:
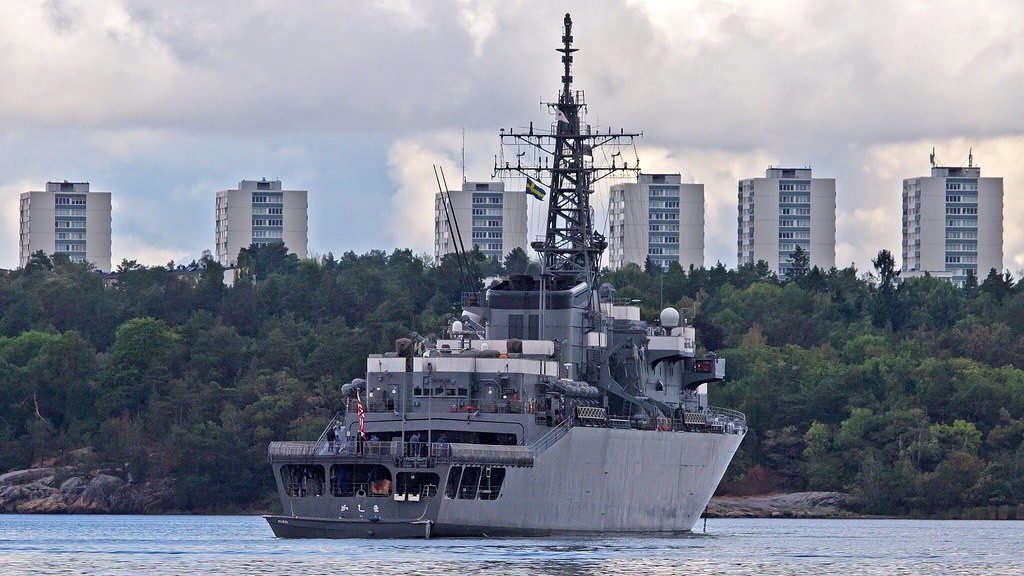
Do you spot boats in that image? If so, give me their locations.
[267,10,753,533]
[262,514,434,539]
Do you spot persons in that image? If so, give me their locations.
[350,436,356,453]
[335,435,342,455]
[368,435,380,454]
[327,427,336,452]
[438,434,447,458]
[356,432,366,455]
[410,432,421,458]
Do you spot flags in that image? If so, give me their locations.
[526,177,546,201]
[357,389,366,440]
